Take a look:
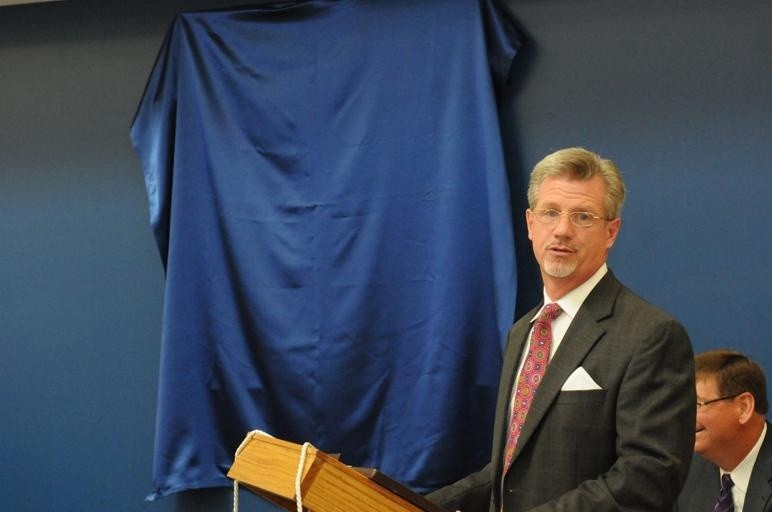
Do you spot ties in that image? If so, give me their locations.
[502,302,564,476]
[713,474,735,512]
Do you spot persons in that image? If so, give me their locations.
[423,146,696,512]
[693,350,772,512]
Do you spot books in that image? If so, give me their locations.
[351,466,446,512]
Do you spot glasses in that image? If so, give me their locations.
[696,393,742,408]
[530,209,605,227]
